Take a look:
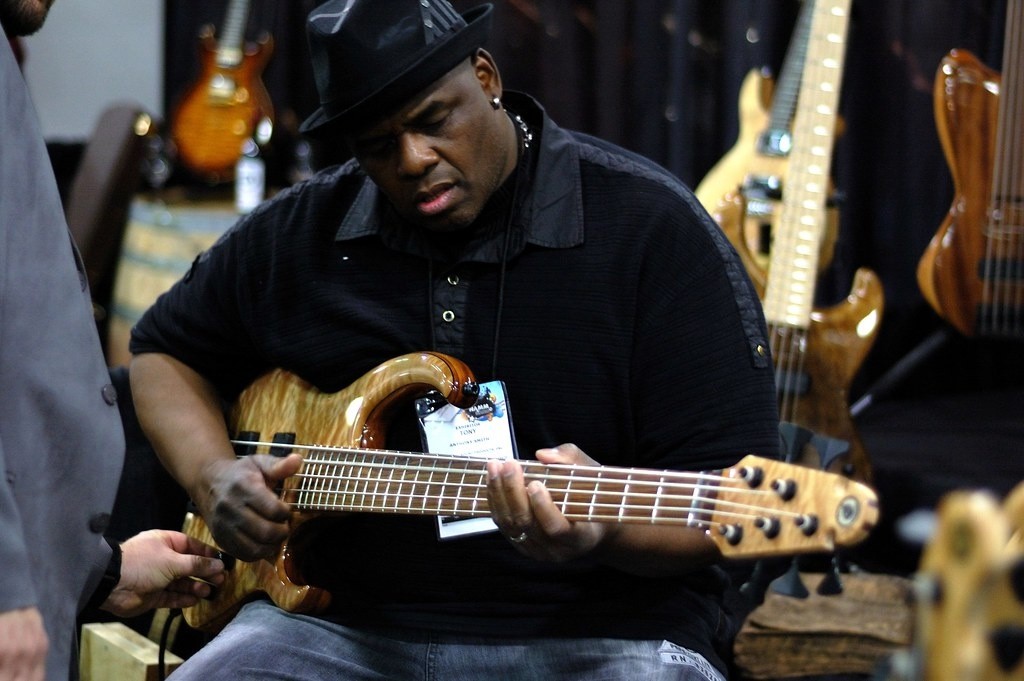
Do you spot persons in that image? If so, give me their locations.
[127,1,783,681]
[0,1,223,681]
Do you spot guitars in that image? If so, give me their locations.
[165,0,278,186]
[174,346,884,630]
[694,0,885,487]
[912,0,1024,346]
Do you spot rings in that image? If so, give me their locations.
[510,533,527,543]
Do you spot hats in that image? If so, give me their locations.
[297,0,494,162]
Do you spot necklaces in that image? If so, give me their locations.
[514,115,533,148]
